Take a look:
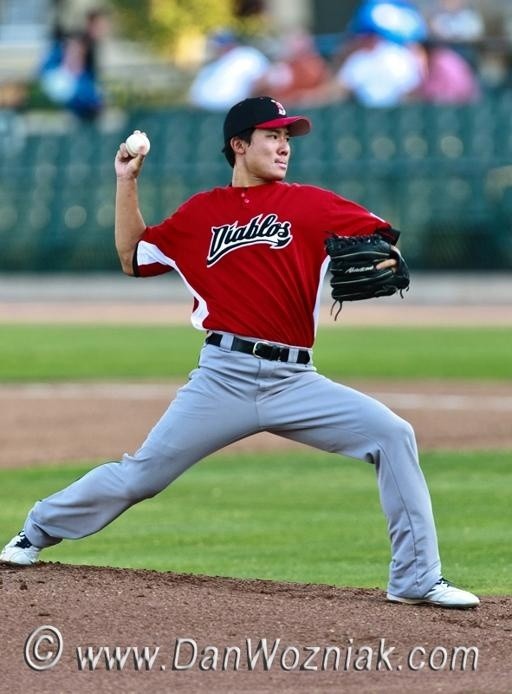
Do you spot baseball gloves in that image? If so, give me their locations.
[325,234,409,300]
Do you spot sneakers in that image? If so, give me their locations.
[0,528,40,566]
[386,577,480,609]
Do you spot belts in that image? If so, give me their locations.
[204,330,311,363]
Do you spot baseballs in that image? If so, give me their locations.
[125,133,150,157]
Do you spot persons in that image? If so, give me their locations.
[0,94,482,611]
[0,0,511,277]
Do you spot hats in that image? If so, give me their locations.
[223,96,311,142]
